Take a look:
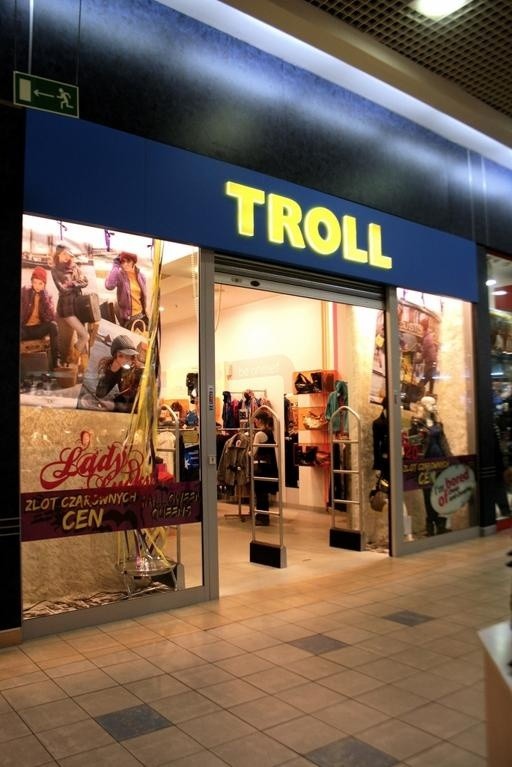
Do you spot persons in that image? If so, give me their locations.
[105,251,150,330]
[415,396,457,534]
[20,265,66,371]
[96,333,146,410]
[51,244,100,363]
[252,411,276,530]
[372,395,413,541]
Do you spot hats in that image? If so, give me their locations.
[110,334,140,356]
[118,252,138,263]
[31,266,47,284]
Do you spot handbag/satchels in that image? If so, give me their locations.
[294,373,314,393]
[302,411,327,430]
[73,289,101,323]
